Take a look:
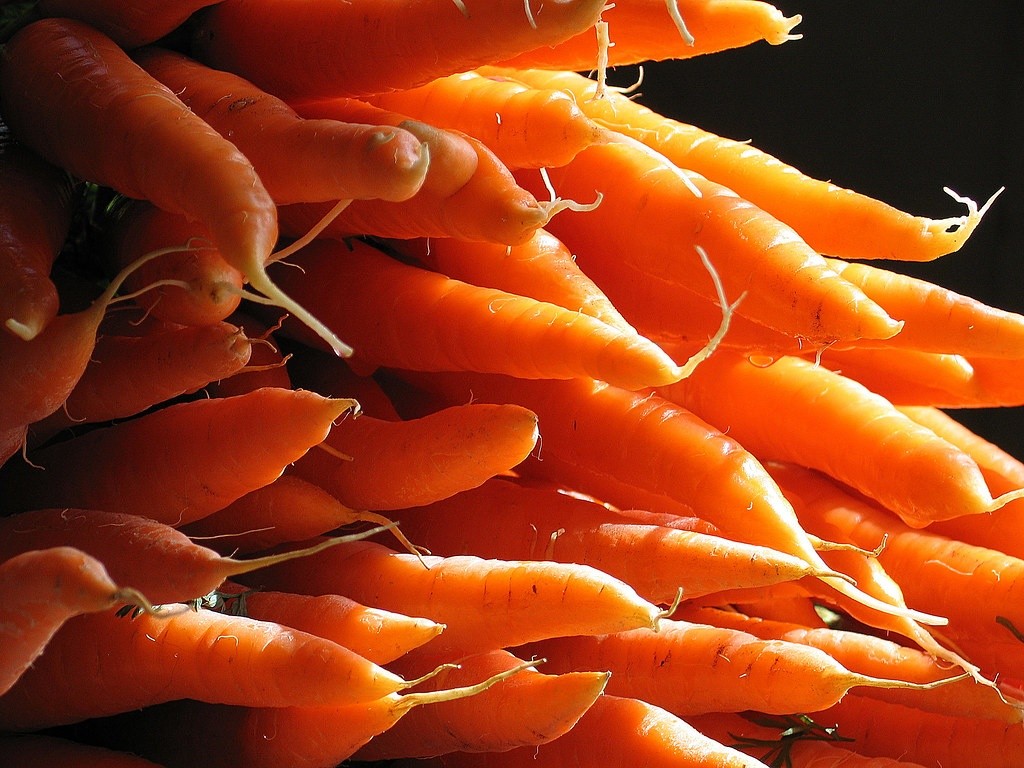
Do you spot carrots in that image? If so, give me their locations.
[0,0,1023,768]
